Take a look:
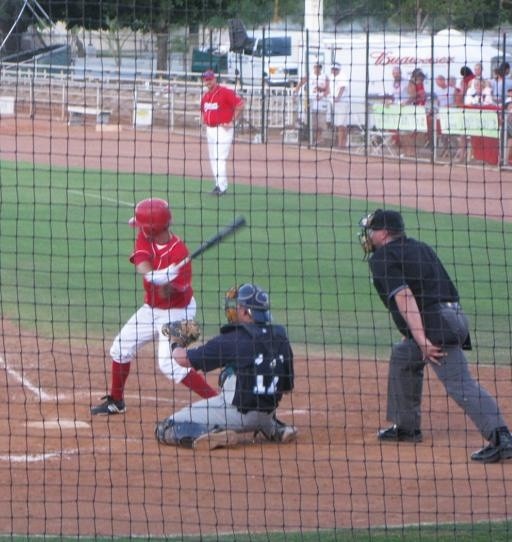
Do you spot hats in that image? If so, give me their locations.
[358,207,408,233]
[225,282,274,324]
[200,67,219,82]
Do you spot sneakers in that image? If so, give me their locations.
[187,429,241,452]
[89,397,130,417]
[274,421,300,443]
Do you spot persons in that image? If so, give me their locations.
[377,61,511,164]
[155,283,299,453]
[88,199,220,415]
[317,62,350,149]
[201,70,245,195]
[356,208,512,463]
[291,61,330,146]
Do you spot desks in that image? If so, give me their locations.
[327,96,512,169]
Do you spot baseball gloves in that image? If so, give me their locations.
[162,320,200,347]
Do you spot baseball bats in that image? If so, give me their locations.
[177,216,246,268]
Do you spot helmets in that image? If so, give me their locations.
[128,195,174,237]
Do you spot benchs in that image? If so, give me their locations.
[65,107,112,127]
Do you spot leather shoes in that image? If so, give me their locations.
[469,431,512,464]
[375,423,426,445]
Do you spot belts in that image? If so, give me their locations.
[421,299,463,315]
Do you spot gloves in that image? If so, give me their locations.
[142,265,181,287]
